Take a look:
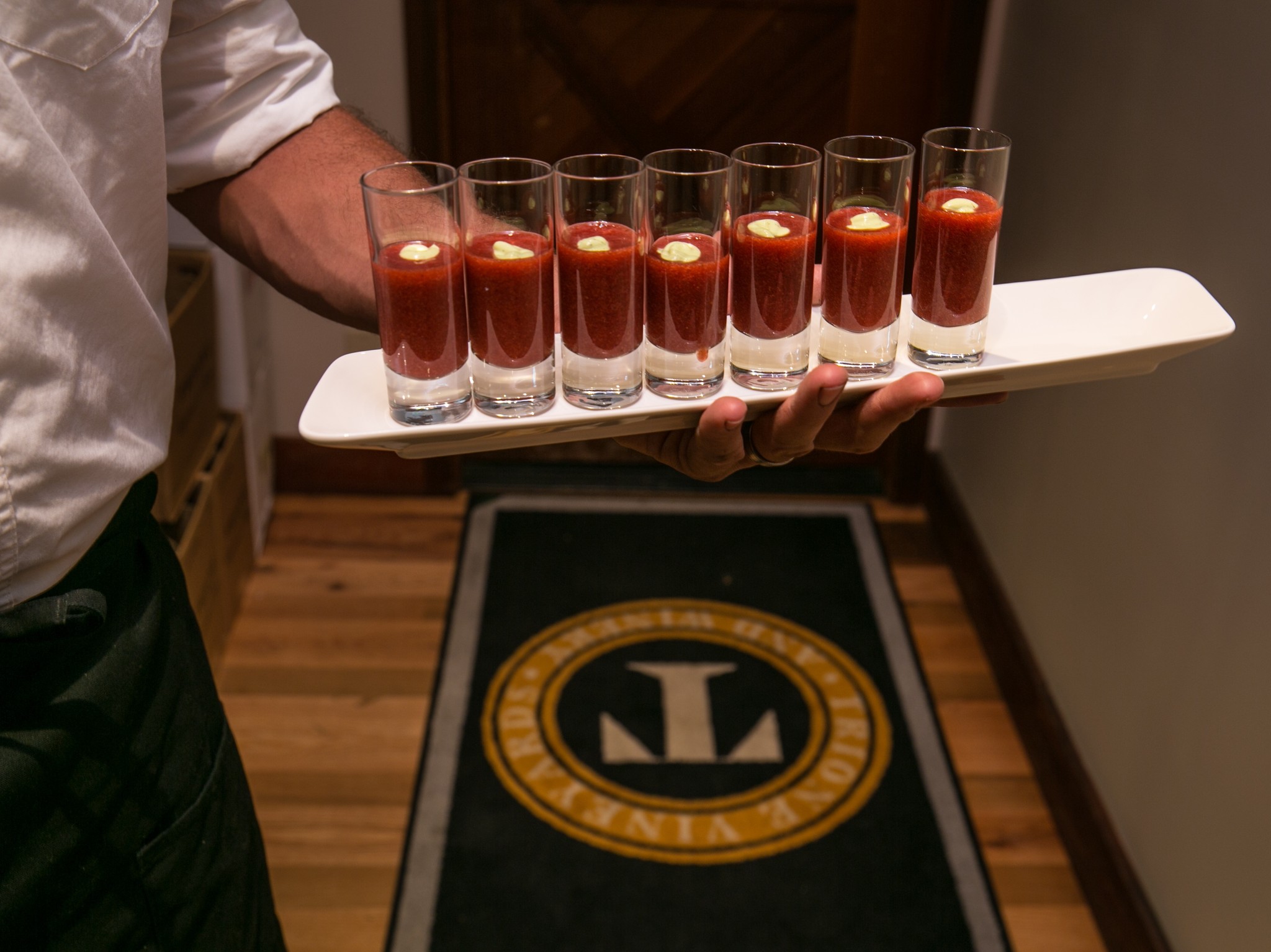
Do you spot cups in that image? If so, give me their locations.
[552,153,647,412]
[818,135,915,384]
[459,156,556,419]
[907,126,1012,372]
[729,141,822,392]
[642,148,735,401]
[359,160,471,426]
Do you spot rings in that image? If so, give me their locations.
[744,425,794,467]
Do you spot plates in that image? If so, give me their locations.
[296,268,1236,458]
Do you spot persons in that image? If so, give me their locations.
[0,0,946,952]
